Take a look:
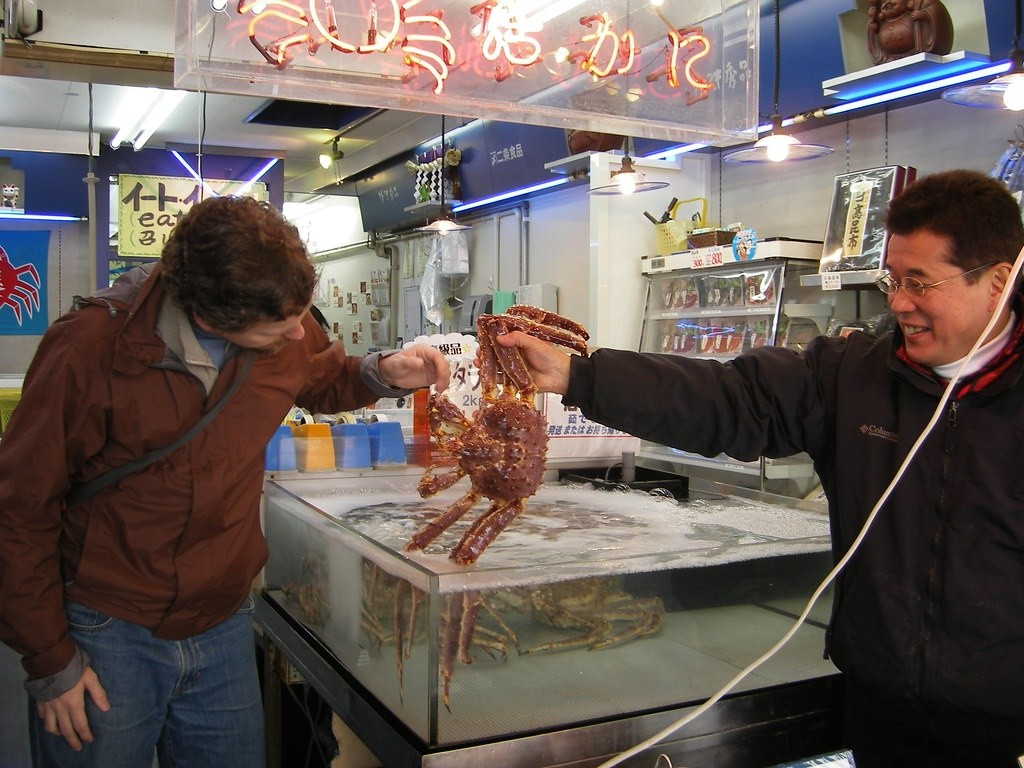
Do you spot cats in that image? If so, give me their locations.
[2,183,20,208]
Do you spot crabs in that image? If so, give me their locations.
[282,303,664,712]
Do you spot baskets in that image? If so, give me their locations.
[687,230,737,248]
[655,197,719,256]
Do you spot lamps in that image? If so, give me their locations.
[413,115,472,235]
[942,0,1024,110]
[110,88,187,152]
[318,140,344,168]
[722,0,837,164]
[472,0,584,44]
[212,0,268,16]
[586,135,670,197]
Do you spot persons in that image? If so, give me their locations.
[864,0,954,66]
[0,197,450,768]
[474,168,1024,768]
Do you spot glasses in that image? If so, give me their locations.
[874,262,995,298]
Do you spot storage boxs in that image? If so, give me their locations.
[819,164,917,273]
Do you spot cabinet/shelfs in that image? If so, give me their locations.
[624,237,886,496]
